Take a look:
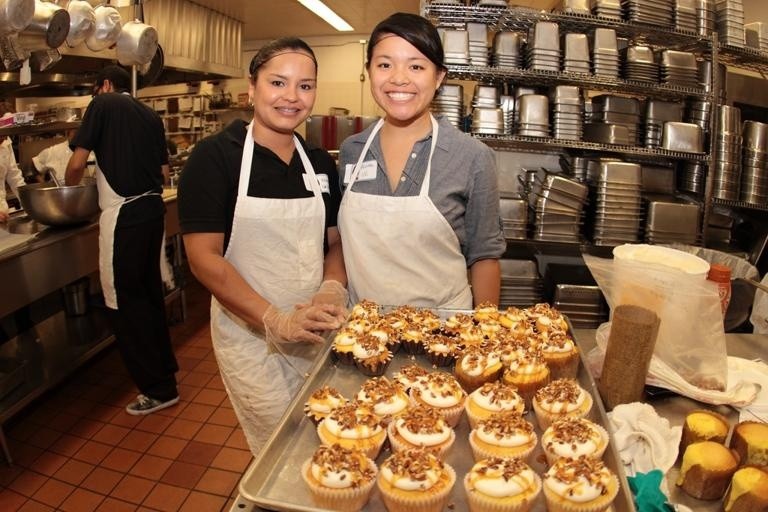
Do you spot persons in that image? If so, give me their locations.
[36,125,97,186]
[0,134,30,226]
[336,11,509,312]
[63,65,182,416]
[177,35,349,459]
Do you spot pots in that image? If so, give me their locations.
[116,0,164,86]
[1,1,38,42]
[63,0,96,50]
[87,1,126,54]
[16,0,71,55]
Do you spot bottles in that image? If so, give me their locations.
[707,260,733,318]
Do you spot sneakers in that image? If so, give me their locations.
[126,393,180,416]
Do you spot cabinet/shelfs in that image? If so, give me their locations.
[420,0,768,268]
[137,95,211,141]
[0,201,187,466]
[0,59,118,136]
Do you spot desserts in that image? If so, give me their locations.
[305,298,620,510]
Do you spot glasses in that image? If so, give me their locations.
[92,86,101,99]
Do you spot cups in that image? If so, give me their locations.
[599,304,664,411]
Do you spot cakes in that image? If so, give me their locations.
[721,465,768,512]
[676,440,738,501]
[679,411,729,458]
[729,420,768,468]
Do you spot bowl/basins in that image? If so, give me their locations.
[667,244,760,332]
[15,175,102,229]
